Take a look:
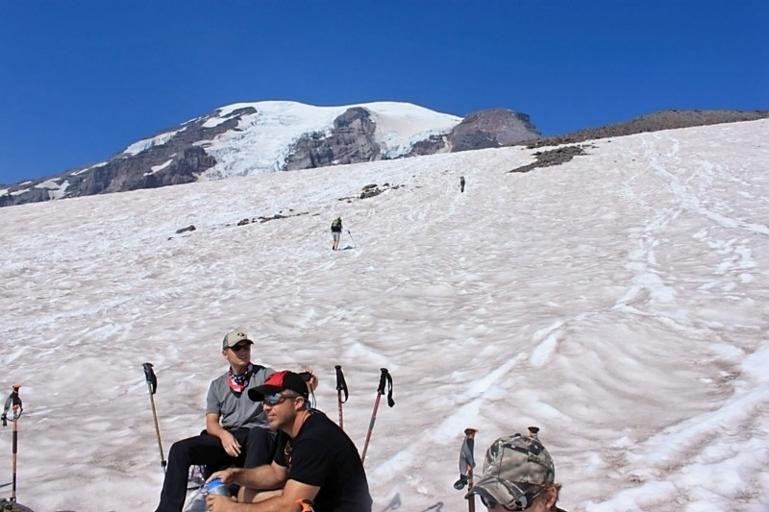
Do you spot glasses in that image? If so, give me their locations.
[261,393,304,406]
[226,344,250,352]
[478,489,545,511]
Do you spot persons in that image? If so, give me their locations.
[156,331,318,512]
[205,371,372,511]
[460,177,465,192]
[465,435,566,512]
[330,217,342,250]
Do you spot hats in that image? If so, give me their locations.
[248,369,310,403]
[464,434,557,503]
[222,329,254,349]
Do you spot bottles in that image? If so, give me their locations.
[206,477,232,498]
